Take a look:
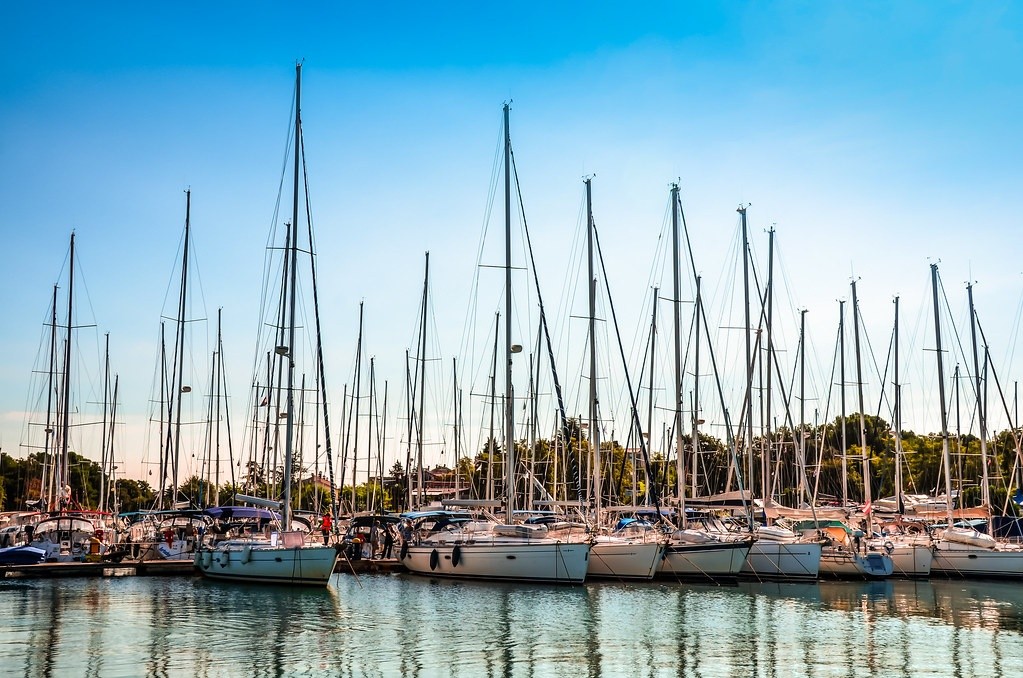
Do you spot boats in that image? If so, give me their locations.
[3,61,1022,602]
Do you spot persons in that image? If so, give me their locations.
[0,469,1023,568]
